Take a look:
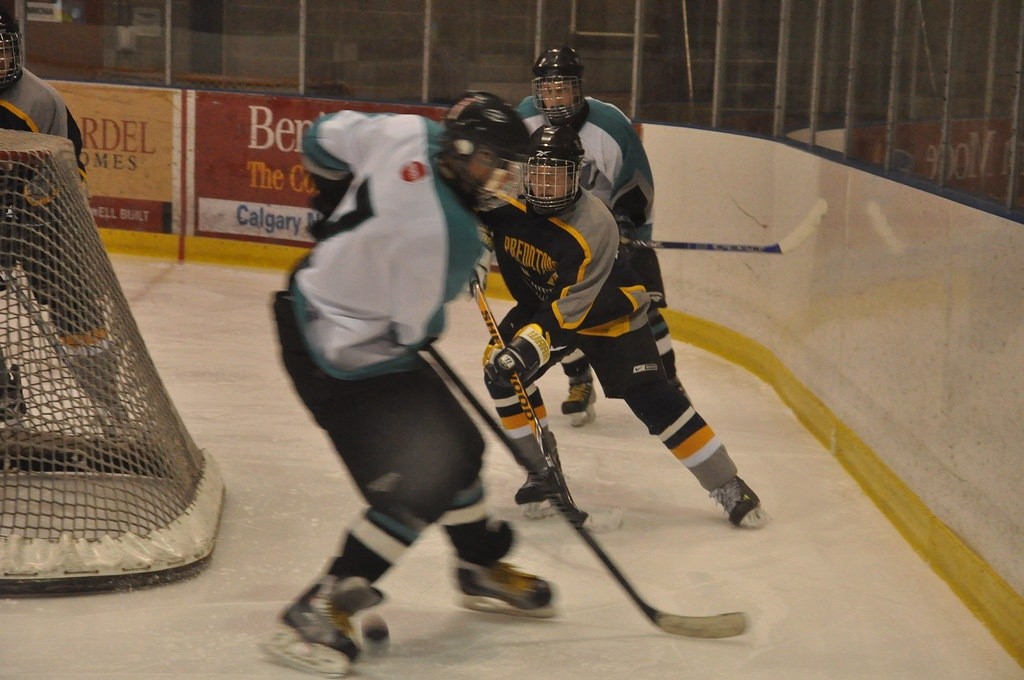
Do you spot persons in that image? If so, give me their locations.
[0,10,127,424]
[465,122,774,530]
[258,90,559,679]
[516,45,688,428]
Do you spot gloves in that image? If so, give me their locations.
[484,328,550,388]
[614,215,638,266]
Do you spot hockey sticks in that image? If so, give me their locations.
[421,343,751,643]
[468,270,630,533]
[6,274,120,440]
[618,196,831,258]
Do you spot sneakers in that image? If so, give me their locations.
[456,563,552,618]
[562,371,596,426]
[262,559,385,676]
[709,475,766,528]
[516,467,558,516]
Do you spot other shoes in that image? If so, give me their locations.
[0,364,24,427]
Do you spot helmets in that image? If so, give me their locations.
[0,11,24,90]
[532,47,586,123]
[433,90,531,212]
[518,124,584,215]
